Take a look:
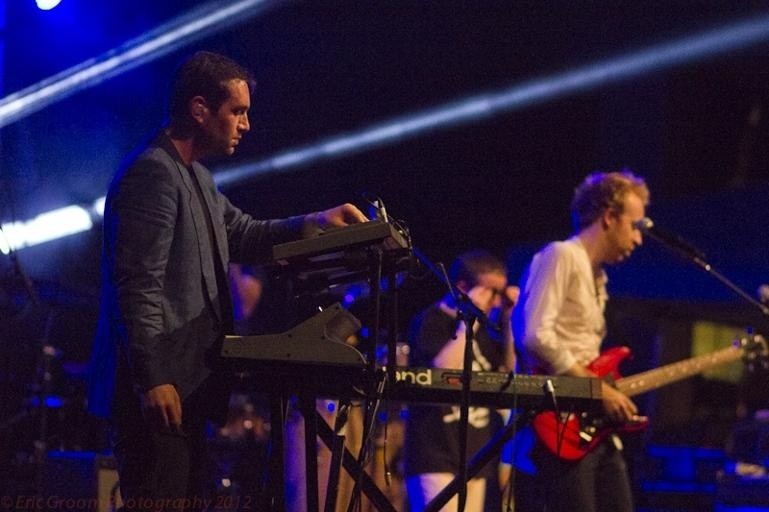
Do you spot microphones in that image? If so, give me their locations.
[638,217,702,266]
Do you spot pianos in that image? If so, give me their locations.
[274,199,411,293]
[219,301,366,398]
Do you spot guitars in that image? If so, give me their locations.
[533,328,768,464]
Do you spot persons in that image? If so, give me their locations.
[497,170,651,512]
[87,52,370,512]
[395,248,517,512]
